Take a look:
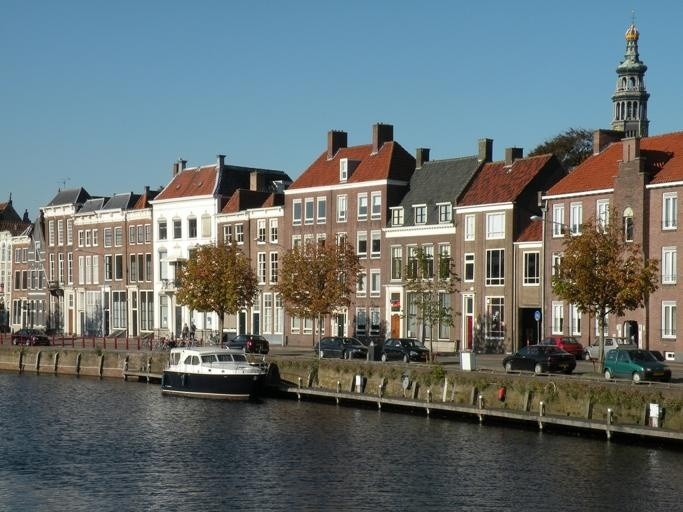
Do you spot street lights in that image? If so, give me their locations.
[528,214,590,343]
[250,236,289,256]
[73,247,105,339]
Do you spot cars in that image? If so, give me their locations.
[501,345,577,376]
[603,347,673,384]
[582,336,636,360]
[314,336,368,359]
[379,337,433,362]
[539,334,584,360]
[9,328,51,346]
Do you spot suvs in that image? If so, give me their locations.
[225,334,271,355]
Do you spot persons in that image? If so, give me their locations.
[183,323,188,340]
[247,336,255,353]
[190,321,198,340]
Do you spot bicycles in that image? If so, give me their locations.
[153,333,201,354]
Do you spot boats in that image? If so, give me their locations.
[159,345,271,402]
